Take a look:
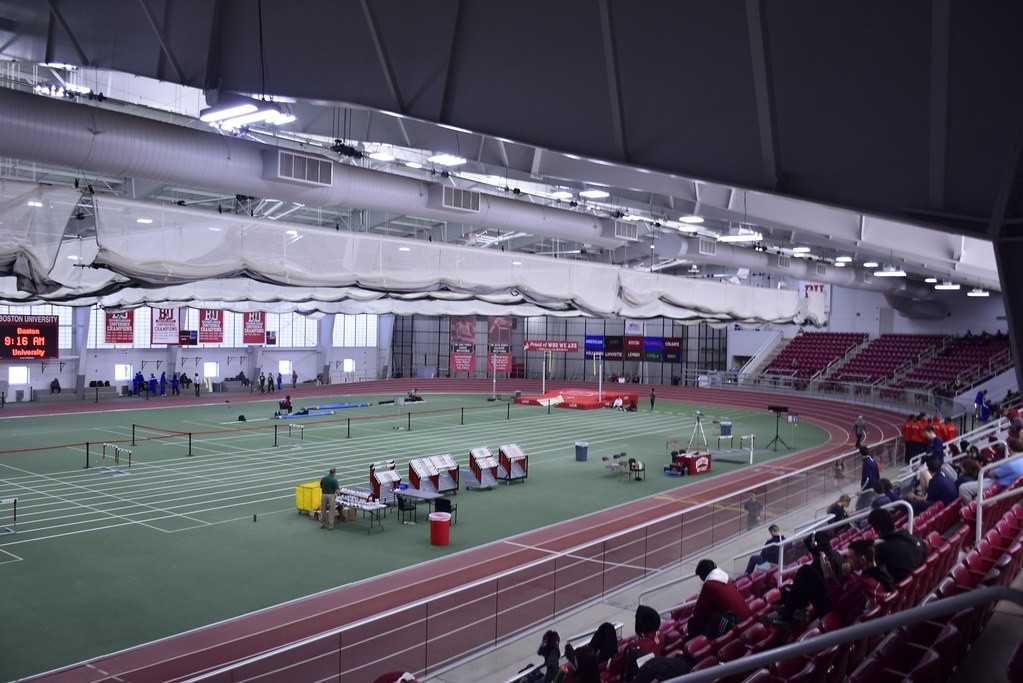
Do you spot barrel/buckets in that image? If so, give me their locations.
[576,442,588,461]
[720,422,732,435]
[429,512,452,546]
[516,391,521,397]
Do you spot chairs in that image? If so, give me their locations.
[713,420,721,436]
[753,331,1013,406]
[396,494,417,525]
[121,386,128,397]
[434,498,457,526]
[597,444,1023,683]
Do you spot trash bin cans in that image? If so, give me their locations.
[429,512,451,546]
[575,441,588,461]
[720,422,731,435]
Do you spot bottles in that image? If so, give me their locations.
[254,514,256,522]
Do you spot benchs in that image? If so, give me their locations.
[142,384,171,398]
[176,383,209,396]
[222,381,251,392]
[33,388,77,402]
[83,386,119,400]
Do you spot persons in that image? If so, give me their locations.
[518,507,927,683]
[650,388,655,411]
[284,396,309,415]
[133,372,200,397]
[743,489,763,530]
[50,378,61,396]
[409,388,417,399]
[489,317,511,334]
[260,370,298,393]
[320,468,340,530]
[808,367,816,376]
[613,396,637,411]
[827,360,1023,532]
[797,327,804,336]
[317,373,323,386]
[452,316,474,343]
[791,360,798,369]
[938,330,1009,356]
[609,372,640,383]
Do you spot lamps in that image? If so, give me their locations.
[717,227,763,243]
[199,88,261,123]
[873,263,907,278]
[934,279,961,291]
[222,99,281,130]
[967,287,990,297]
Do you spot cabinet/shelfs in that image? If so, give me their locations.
[369,459,402,508]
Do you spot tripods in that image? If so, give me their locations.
[765,413,790,452]
[687,411,709,453]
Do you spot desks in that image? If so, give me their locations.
[388,488,444,522]
[676,452,712,475]
[335,499,386,536]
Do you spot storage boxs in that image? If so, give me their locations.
[308,510,318,521]
[664,470,681,478]
[296,480,322,511]
[317,510,340,521]
[664,465,688,476]
[342,507,356,521]
